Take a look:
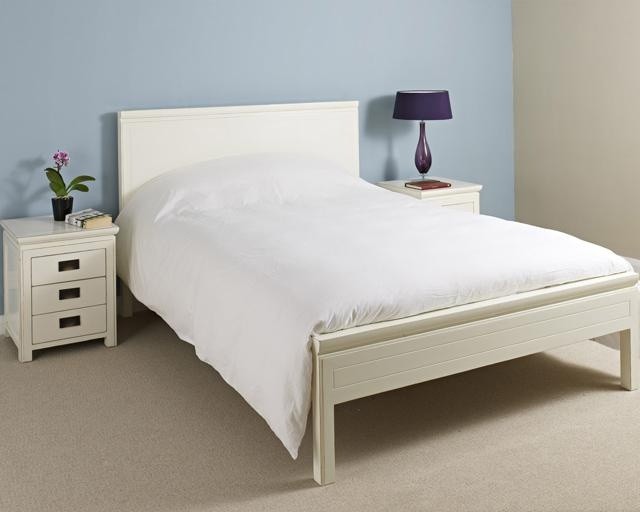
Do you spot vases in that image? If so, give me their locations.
[52,196,73,221]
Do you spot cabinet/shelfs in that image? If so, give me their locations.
[376,177,482,214]
[1,215,119,362]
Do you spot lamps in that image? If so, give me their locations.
[392,89,452,180]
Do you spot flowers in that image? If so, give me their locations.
[43,148,95,198]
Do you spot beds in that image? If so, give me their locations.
[112,99,639,487]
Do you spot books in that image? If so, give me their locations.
[65,207,113,230]
[405,178,452,190]
